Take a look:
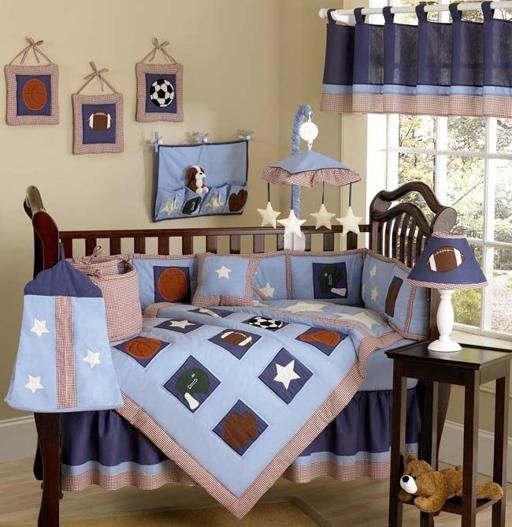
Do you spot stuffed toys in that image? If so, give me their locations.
[395,453,505,514]
[186,164,209,195]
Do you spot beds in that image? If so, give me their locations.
[23,181,452,527]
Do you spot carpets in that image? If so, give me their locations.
[14,495,333,527]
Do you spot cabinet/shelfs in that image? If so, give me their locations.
[383,338,512,527]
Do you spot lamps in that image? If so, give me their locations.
[405,231,488,352]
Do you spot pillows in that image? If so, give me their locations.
[132,248,429,341]
